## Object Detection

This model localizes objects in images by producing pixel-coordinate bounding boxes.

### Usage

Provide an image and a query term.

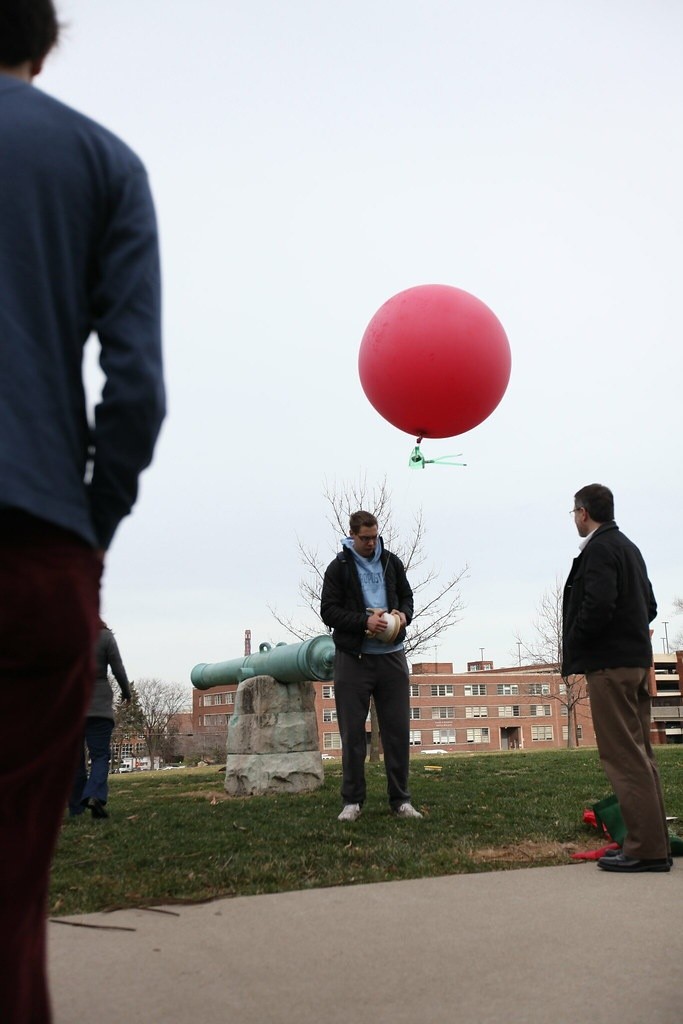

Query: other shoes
[91,802,108,820]
[596,854,670,872]
[605,849,673,866]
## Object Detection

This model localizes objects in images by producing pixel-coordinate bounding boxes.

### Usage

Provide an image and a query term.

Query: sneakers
[397,802,423,819]
[338,803,361,822]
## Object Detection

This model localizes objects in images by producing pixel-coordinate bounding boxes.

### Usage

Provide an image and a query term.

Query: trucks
[118,756,186,773]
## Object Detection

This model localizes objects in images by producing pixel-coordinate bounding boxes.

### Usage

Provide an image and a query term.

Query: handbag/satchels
[592,794,628,848]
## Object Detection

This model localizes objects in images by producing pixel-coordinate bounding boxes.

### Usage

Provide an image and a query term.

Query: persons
[560,484,673,872]
[67,617,131,818]
[0,0,167,1023]
[321,510,424,821]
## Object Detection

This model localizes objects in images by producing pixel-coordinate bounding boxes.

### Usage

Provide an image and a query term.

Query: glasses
[572,505,587,517]
[354,531,377,541]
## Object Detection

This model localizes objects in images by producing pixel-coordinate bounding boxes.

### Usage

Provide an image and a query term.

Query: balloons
[359,284,511,443]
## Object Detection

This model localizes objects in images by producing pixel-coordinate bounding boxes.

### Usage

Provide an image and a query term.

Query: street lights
[660,638,666,654]
[662,621,670,654]
[479,648,485,670]
[516,642,523,667]
